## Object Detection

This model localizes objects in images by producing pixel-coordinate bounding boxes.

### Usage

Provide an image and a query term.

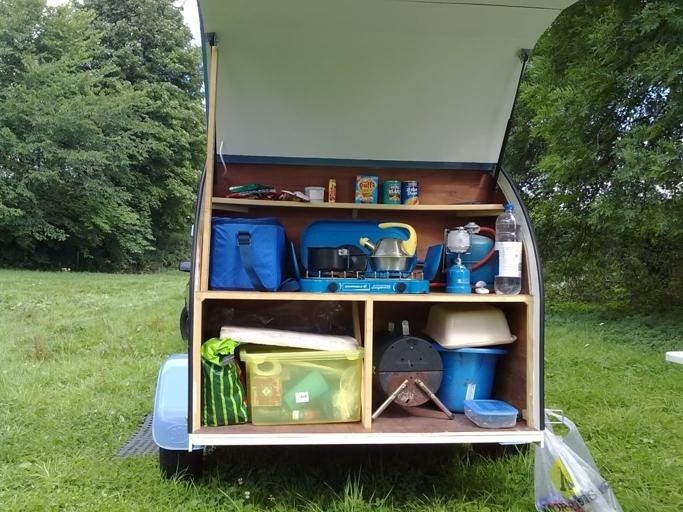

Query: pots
[305,246,350,273]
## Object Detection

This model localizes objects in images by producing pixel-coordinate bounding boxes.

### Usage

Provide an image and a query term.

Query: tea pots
[360,221,417,272]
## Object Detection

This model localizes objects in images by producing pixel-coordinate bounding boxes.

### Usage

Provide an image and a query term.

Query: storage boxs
[239,349,365,427]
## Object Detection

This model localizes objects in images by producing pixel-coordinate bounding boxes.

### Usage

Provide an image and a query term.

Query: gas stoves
[299,268,430,295]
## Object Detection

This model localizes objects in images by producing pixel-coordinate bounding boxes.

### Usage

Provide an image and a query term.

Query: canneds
[400,181,419,205]
[383,180,402,204]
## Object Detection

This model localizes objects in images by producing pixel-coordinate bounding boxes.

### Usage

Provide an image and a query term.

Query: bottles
[326,175,337,203]
[252,407,299,423]
[491,200,523,295]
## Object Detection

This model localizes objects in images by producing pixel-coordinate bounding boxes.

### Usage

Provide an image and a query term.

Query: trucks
[149,0,578,478]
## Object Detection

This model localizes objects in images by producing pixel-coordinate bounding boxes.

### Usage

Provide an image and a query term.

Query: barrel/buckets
[431,343,506,414]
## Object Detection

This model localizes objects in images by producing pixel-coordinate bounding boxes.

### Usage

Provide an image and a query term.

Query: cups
[282,371,329,409]
[303,186,325,202]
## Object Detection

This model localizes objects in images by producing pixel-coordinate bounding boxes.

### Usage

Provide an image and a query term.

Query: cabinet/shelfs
[188,157,549,446]
